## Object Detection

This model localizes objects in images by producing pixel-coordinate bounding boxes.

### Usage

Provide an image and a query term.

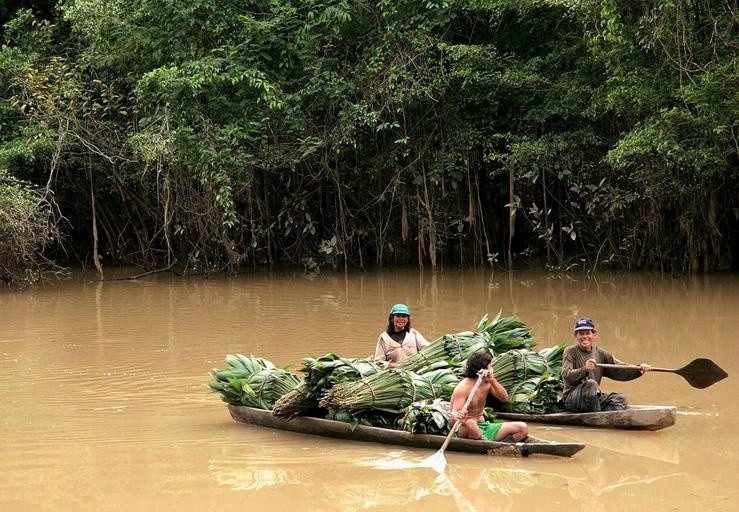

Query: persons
[374,303,431,368]
[448,351,528,442]
[562,317,651,412]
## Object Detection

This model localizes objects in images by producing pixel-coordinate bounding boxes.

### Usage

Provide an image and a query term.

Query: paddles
[591,358,729,389]
[420,370,490,468]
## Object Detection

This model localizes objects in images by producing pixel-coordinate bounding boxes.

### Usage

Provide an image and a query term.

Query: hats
[575,317,595,331]
[390,304,410,315]
